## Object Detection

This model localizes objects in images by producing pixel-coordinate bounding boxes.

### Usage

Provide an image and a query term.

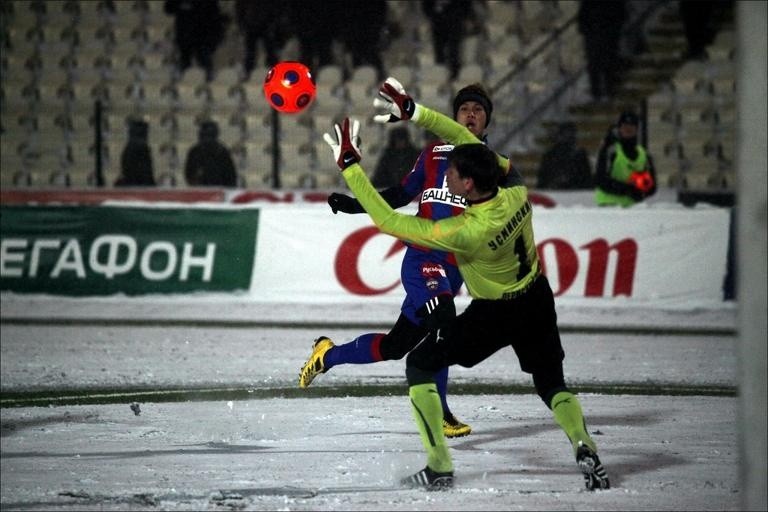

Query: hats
[617,110,639,126]
[452,81,493,131]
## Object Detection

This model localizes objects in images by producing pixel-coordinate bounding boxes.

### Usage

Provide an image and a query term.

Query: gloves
[327,186,362,220]
[322,115,364,173]
[370,75,416,125]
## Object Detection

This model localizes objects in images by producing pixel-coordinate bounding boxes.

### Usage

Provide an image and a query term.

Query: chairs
[0,0,587,191]
[647,28,736,196]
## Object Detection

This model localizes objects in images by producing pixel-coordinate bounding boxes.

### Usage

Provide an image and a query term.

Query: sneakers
[440,417,473,440]
[399,464,455,491]
[296,335,336,391]
[574,443,611,492]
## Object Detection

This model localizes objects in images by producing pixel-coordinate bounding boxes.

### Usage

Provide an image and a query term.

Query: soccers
[263,60,316,115]
[627,170,656,193]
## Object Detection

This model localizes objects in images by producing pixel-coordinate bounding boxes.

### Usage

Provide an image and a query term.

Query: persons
[298,85,493,438]
[322,76,610,491]
